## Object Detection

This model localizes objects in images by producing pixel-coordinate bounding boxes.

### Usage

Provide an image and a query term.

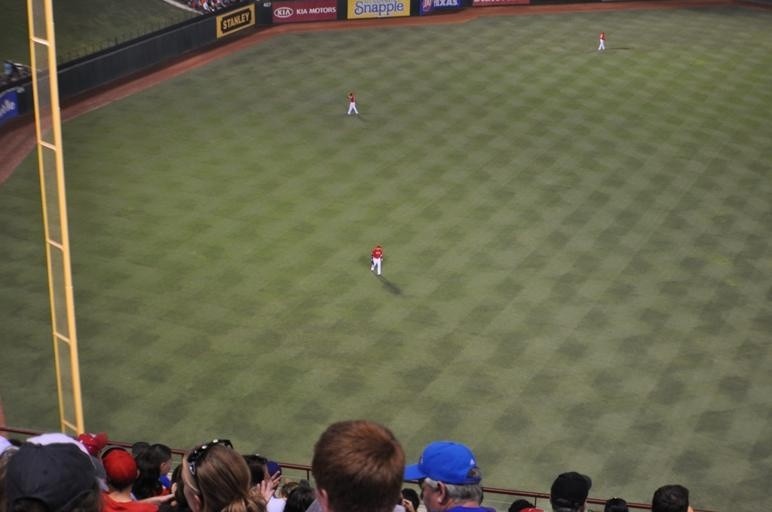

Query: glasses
[187,438,233,504]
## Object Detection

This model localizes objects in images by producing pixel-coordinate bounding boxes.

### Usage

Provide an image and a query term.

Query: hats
[102,448,137,483]
[404,441,482,485]
[6,433,107,511]
[78,432,108,455]
[266,461,281,478]
[551,474,591,507]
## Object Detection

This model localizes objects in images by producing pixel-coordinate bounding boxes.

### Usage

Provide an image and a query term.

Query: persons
[189,1,243,15]
[347,92,359,116]
[370,245,383,275]
[598,32,606,51]
